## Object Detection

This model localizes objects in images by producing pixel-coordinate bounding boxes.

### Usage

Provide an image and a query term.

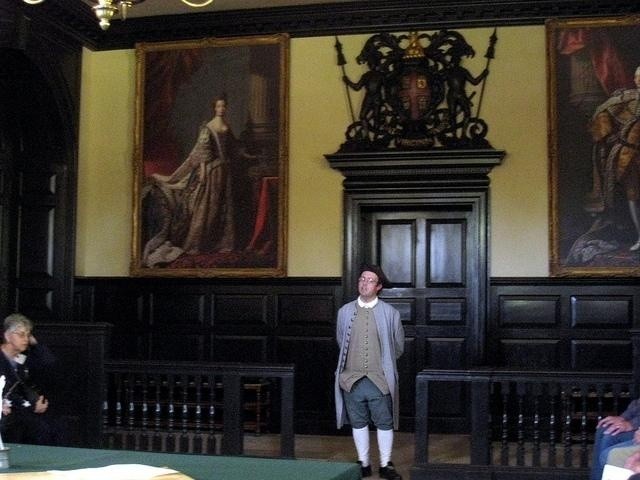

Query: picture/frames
[130,33,290,279]
[544,15,640,278]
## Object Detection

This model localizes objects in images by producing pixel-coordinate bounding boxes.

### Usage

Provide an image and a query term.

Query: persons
[590,399,640,480]
[559,68,640,267]
[432,56,489,146]
[343,55,394,145]
[334,265,405,480]
[138,94,271,268]
[1,315,70,447]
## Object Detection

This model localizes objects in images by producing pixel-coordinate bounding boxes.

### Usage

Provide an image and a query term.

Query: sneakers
[379,461,402,479]
[356,460,372,477]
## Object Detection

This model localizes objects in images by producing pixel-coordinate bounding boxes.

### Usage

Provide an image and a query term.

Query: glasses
[359,276,374,283]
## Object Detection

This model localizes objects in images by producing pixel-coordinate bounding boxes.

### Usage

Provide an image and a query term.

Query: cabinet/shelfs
[123,379,270,437]
[559,390,631,445]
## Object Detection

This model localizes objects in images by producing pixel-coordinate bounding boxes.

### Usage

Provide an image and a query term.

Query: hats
[360,265,384,282]
